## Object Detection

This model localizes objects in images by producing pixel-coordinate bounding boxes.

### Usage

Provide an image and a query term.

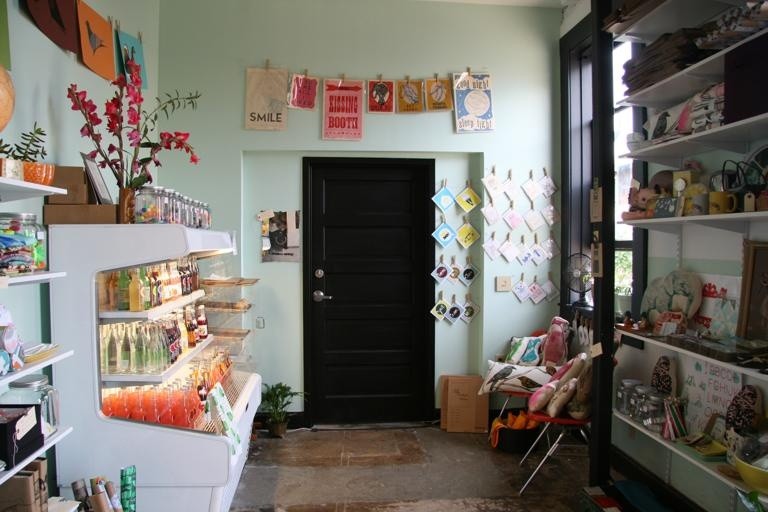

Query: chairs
[488,329,548,440]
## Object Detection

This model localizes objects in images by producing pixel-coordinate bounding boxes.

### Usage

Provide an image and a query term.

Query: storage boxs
[40,165,119,228]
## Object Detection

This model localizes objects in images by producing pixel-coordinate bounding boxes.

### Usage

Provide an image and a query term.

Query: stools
[513,406,590,495]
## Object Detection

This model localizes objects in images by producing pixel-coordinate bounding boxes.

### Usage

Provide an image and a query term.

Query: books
[0,457,49,511]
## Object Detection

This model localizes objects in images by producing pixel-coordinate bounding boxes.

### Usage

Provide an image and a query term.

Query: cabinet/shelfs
[1,177,82,512]
[45,221,265,511]
[597,0,768,511]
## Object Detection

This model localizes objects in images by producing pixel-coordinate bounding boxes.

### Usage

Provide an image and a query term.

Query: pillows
[477,317,589,417]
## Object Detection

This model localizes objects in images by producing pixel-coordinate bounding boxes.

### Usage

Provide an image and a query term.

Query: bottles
[101,345,232,427]
[616,378,672,432]
[134,185,212,229]
[0,374,60,439]
[108,256,203,312]
[100,305,214,373]
[0,210,47,269]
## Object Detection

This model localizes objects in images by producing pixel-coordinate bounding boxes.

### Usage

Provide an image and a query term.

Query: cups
[708,190,737,215]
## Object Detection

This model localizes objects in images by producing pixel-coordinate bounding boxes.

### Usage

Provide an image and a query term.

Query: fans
[561,248,594,306]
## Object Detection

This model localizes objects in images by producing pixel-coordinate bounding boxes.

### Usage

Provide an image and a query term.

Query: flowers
[66,54,203,195]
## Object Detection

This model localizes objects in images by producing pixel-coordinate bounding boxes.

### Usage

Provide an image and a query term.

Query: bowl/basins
[732,451,768,496]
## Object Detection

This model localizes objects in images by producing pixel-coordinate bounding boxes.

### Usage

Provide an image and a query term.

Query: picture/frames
[730,234,768,350]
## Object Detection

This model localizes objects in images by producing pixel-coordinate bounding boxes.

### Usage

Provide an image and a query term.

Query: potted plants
[256,378,310,436]
[0,117,57,185]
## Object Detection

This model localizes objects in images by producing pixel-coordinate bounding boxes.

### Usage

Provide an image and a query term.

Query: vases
[118,192,135,224]
